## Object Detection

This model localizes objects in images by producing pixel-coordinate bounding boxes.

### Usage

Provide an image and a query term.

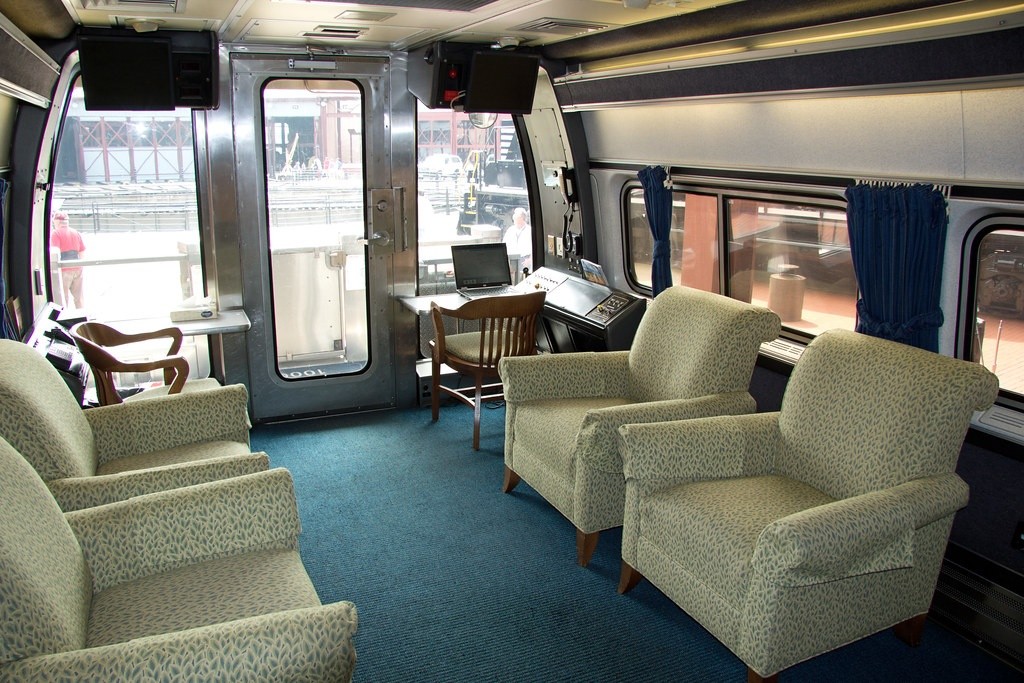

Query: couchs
[1,337,273,514]
[496,281,781,575]
[616,327,1001,681]
[1,431,360,683]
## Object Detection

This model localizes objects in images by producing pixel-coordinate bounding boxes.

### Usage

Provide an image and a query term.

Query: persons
[51,212,86,310]
[502,208,533,274]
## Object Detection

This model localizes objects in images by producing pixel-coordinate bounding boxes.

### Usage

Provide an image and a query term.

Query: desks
[164,308,254,384]
[398,285,524,321]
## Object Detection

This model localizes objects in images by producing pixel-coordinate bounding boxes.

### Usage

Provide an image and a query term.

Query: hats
[52,210,70,220]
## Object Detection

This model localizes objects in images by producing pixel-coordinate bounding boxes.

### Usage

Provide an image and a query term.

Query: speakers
[81,28,219,108]
[407,41,534,109]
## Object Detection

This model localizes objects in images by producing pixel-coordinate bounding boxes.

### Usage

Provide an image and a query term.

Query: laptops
[451,242,527,302]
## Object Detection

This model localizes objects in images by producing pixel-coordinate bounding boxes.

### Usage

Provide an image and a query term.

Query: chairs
[68,320,252,429]
[425,290,555,455]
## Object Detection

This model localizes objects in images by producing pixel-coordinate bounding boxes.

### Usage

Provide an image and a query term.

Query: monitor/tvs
[78,38,172,111]
[464,48,540,114]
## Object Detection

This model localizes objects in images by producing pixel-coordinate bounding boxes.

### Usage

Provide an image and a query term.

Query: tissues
[171,294,220,323]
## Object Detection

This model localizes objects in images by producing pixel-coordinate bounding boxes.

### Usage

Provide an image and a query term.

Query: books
[580,259,608,286]
[5,296,24,341]
